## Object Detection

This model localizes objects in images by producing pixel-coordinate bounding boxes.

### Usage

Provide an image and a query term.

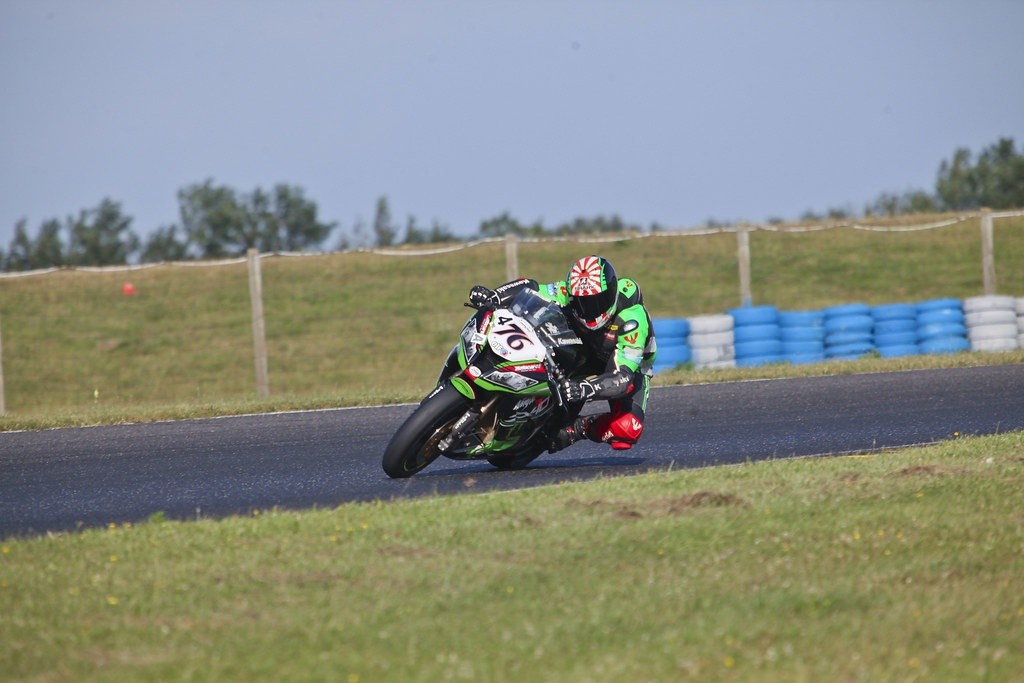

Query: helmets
[567,255,617,330]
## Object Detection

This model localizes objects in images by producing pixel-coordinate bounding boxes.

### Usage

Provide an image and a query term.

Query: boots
[554,412,608,449]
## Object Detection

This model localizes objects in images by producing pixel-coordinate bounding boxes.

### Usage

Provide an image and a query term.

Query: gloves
[469,286,498,311]
[560,378,594,403]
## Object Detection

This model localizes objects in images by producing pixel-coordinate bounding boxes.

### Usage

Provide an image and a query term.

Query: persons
[470,254,657,455]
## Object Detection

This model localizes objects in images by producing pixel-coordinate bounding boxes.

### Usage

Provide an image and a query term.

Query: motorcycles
[380,277,596,481]
